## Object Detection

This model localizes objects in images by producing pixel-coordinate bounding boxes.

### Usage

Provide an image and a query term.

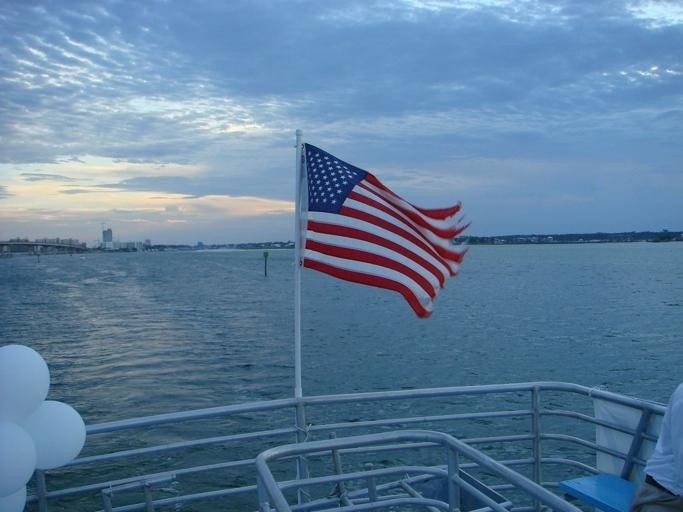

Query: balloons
[0,421,37,497]
[0,486,27,512]
[22,401,86,470]
[0,344,50,422]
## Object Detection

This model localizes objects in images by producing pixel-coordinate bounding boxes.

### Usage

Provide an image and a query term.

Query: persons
[630,383,683,512]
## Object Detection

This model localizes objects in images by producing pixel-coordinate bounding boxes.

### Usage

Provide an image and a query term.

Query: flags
[298,143,473,319]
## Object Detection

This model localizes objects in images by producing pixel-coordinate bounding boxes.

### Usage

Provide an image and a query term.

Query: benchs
[558,473,637,512]
